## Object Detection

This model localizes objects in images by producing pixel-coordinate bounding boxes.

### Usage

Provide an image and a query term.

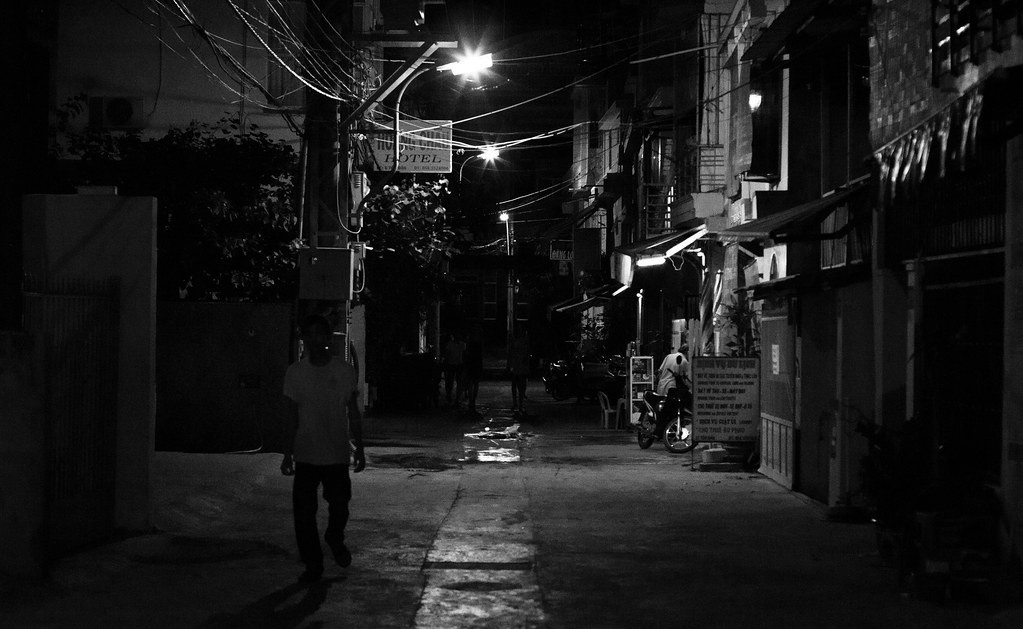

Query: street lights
[343,34,499,361]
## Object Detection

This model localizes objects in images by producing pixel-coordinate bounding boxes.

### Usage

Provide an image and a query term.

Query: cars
[479,335,511,379]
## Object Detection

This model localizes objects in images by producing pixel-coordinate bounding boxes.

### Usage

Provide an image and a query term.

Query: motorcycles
[543,347,630,405]
[631,356,699,453]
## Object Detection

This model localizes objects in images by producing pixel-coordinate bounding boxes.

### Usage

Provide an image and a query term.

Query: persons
[455,335,481,415]
[439,332,466,410]
[509,324,529,412]
[657,343,691,397]
[281,314,366,581]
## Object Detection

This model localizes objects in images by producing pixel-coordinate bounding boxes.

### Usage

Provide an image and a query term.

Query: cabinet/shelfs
[629,355,655,424]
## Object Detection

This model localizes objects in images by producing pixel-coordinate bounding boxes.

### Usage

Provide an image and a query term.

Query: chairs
[597,391,617,429]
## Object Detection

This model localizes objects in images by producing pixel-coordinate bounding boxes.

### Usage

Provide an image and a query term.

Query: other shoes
[298,565,324,582]
[330,543,352,568]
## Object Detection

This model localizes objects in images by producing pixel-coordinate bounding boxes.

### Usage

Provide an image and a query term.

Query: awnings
[616,223,708,258]
[716,179,870,242]
[556,295,612,313]
[732,273,802,293]
